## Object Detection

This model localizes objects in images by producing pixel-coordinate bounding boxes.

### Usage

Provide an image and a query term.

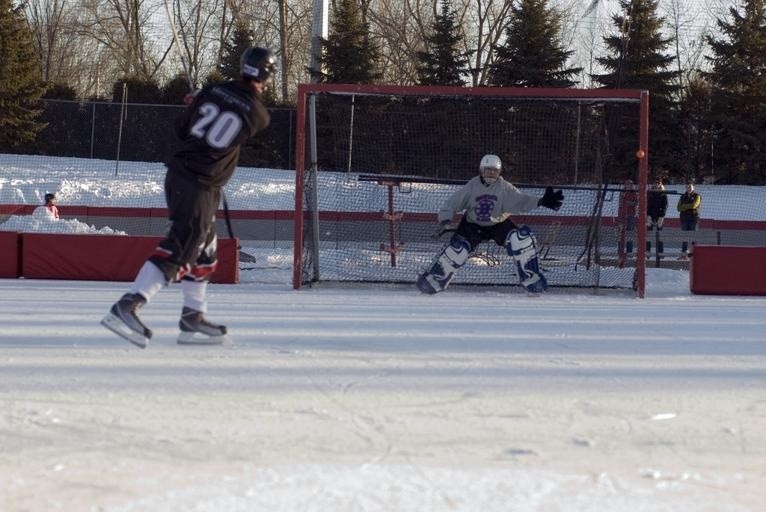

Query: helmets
[239,47,279,83]
[45,194,55,201]
[480,155,502,185]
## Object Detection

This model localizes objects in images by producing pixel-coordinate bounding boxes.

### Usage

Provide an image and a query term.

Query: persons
[109,47,282,340]
[645,175,669,258]
[41,194,61,220]
[417,154,564,296]
[617,179,639,257]
[677,180,702,259]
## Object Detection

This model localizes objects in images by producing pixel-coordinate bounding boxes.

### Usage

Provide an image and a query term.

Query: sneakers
[179,306,226,336]
[111,293,152,339]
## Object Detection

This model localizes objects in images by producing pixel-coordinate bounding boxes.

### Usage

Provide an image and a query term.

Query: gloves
[432,220,458,240]
[537,186,564,212]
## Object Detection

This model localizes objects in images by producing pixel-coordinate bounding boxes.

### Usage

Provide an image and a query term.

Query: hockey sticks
[222,187,256,262]
[577,182,608,266]
